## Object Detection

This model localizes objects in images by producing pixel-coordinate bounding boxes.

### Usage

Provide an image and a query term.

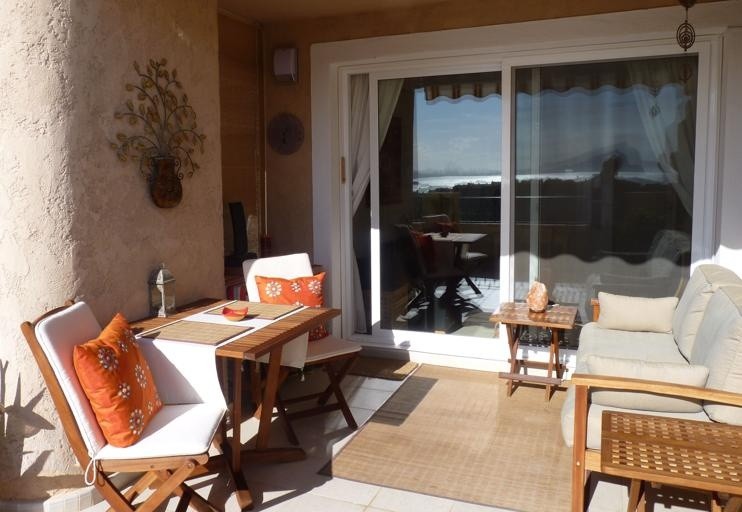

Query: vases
[151,157,182,208]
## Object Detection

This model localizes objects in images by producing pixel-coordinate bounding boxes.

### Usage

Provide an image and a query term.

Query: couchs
[585,229,691,323]
[560,263,742,512]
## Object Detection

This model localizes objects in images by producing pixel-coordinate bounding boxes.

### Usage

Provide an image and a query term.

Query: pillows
[588,356,709,413]
[596,291,679,334]
[255,271,329,342]
[73,313,163,449]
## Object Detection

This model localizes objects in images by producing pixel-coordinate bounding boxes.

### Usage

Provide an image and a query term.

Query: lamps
[272,47,298,82]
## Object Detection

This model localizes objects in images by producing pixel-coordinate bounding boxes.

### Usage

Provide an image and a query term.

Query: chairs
[423,214,487,294]
[242,251,363,456]
[21,297,228,512]
[393,224,463,309]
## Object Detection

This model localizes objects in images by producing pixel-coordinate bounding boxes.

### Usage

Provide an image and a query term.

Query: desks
[426,232,487,298]
[489,302,578,402]
[601,409,742,510]
[127,298,343,512]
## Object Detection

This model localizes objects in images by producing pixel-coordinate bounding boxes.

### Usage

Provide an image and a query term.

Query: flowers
[110,57,207,179]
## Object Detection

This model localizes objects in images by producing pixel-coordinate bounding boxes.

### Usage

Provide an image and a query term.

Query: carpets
[317,362,592,512]
[350,356,419,381]
